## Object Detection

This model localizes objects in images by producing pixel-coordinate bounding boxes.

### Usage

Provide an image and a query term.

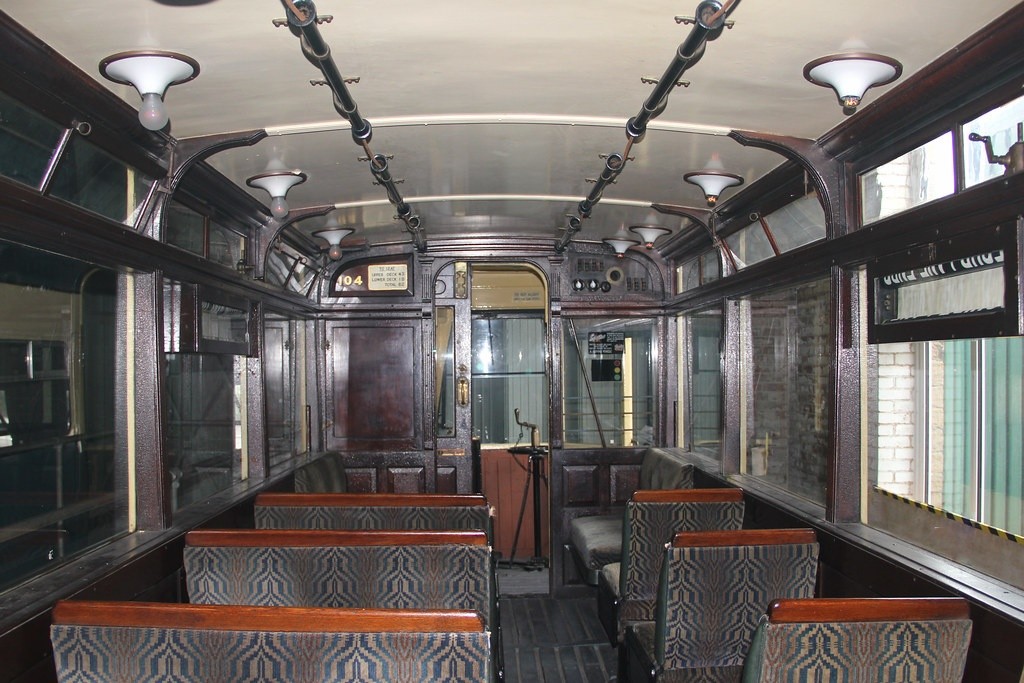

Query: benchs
[183,527,500,662]
[50,599,501,683]
[252,490,495,557]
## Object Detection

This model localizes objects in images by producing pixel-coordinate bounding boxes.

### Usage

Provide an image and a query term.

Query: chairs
[569,444,695,586]
[741,597,972,683]
[597,485,746,649]
[616,527,820,683]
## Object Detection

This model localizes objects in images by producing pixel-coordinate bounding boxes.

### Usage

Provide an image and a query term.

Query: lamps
[803,51,904,116]
[604,237,642,259]
[98,49,201,131]
[629,224,673,250]
[246,170,308,219]
[683,170,744,208]
[311,227,356,261]
